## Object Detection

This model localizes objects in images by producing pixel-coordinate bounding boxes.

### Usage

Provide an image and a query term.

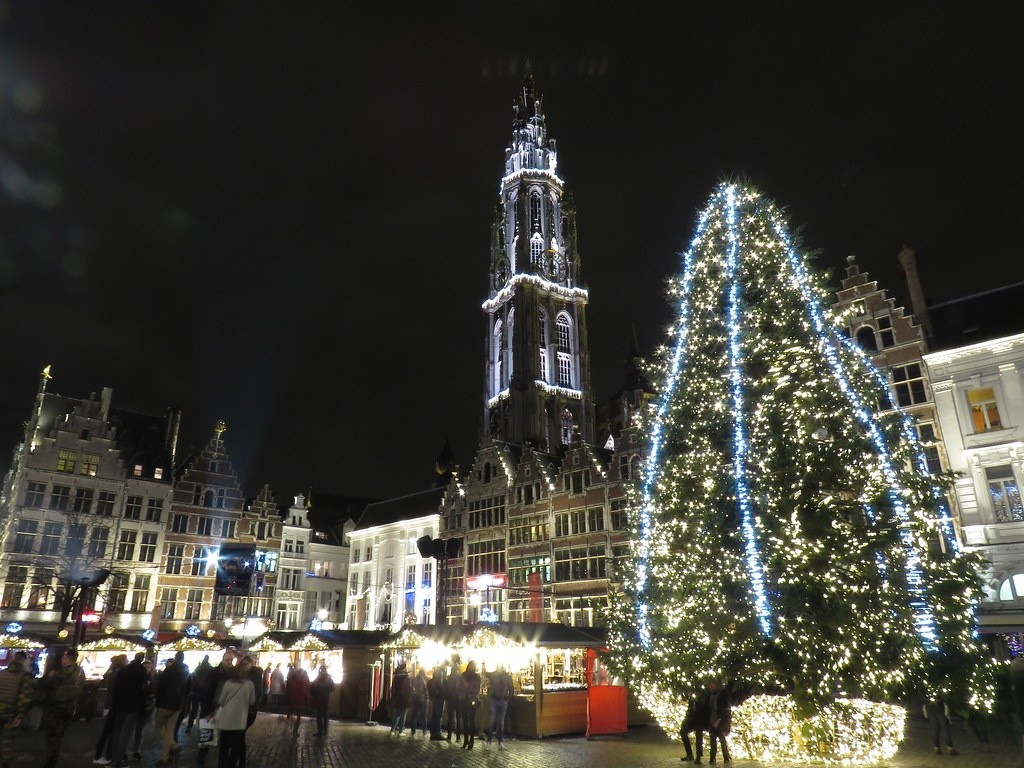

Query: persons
[0,650,85,768]
[390,661,481,750]
[310,666,335,736]
[922,695,955,754]
[680,679,732,764]
[969,710,991,752]
[481,656,514,751]
[92,650,310,768]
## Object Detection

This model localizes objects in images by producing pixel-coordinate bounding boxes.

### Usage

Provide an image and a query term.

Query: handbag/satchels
[468,695,482,711]
[197,715,218,748]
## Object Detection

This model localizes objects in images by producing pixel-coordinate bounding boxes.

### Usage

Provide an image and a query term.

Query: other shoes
[467,740,474,749]
[92,757,112,765]
[133,753,144,761]
[398,732,407,737]
[456,734,461,742]
[695,755,700,764]
[948,748,960,755]
[498,742,507,750]
[724,756,731,763]
[313,729,327,736]
[935,748,942,755]
[461,739,471,748]
[681,754,694,761]
[430,733,446,741]
[446,734,451,741]
[709,757,716,764]
[485,743,491,750]
[389,731,395,736]
